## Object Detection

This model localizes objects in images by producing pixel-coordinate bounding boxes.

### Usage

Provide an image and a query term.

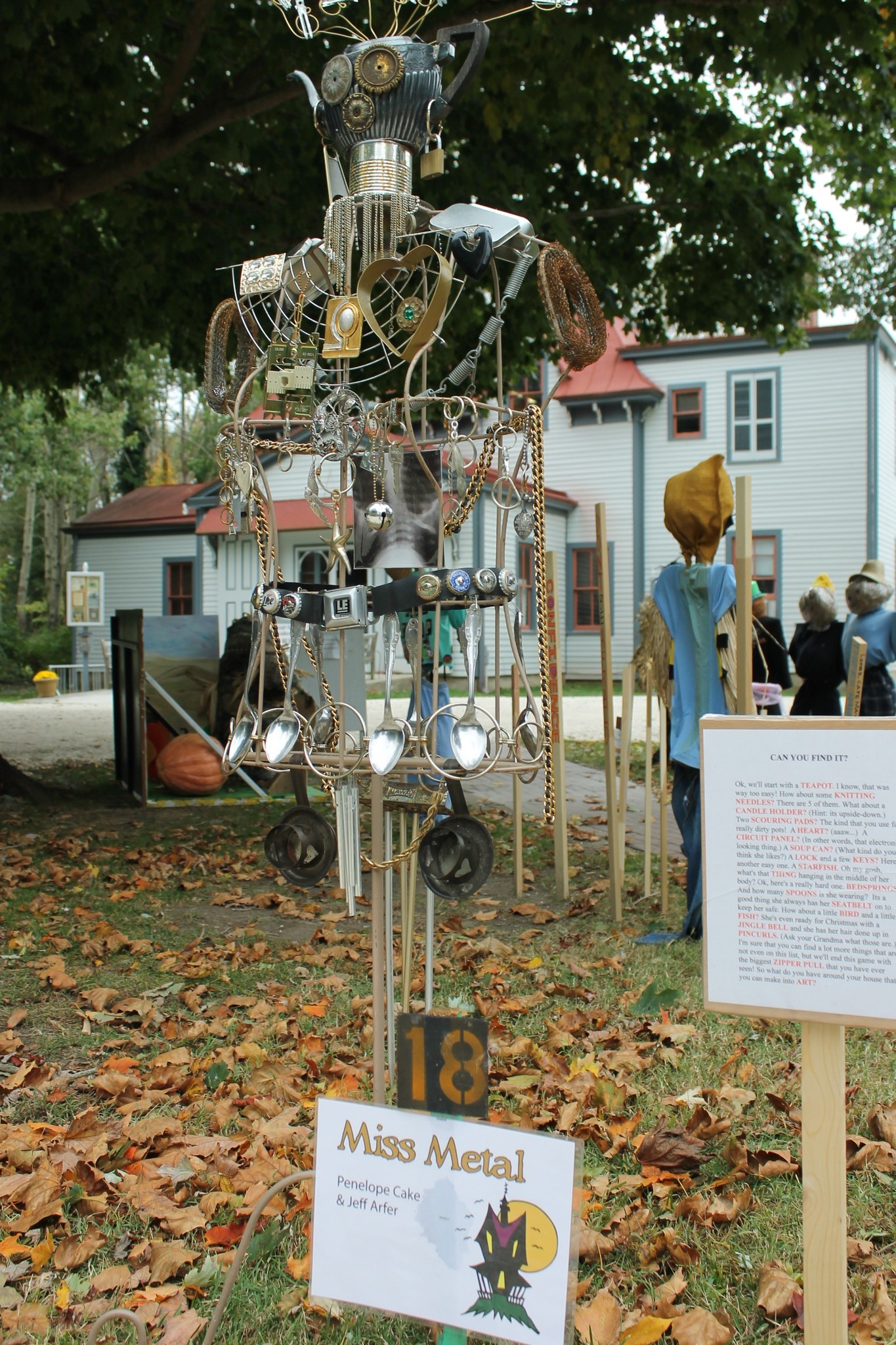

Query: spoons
[450,605,488,769]
[402,618,432,759]
[264,589,306,763]
[368,610,405,776]
[457,620,490,759]
[228,610,262,763]
[513,611,537,760]
[310,622,340,755]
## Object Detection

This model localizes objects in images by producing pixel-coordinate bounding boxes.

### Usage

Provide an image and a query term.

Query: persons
[632,456,755,945]
[751,580,793,715]
[788,574,848,716]
[840,559,896,716]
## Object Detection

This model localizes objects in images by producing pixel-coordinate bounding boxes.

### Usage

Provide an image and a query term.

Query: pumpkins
[156,734,229,794]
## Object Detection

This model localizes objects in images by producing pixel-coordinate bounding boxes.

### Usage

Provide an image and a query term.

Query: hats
[849,559,887,584]
[751,580,766,601]
